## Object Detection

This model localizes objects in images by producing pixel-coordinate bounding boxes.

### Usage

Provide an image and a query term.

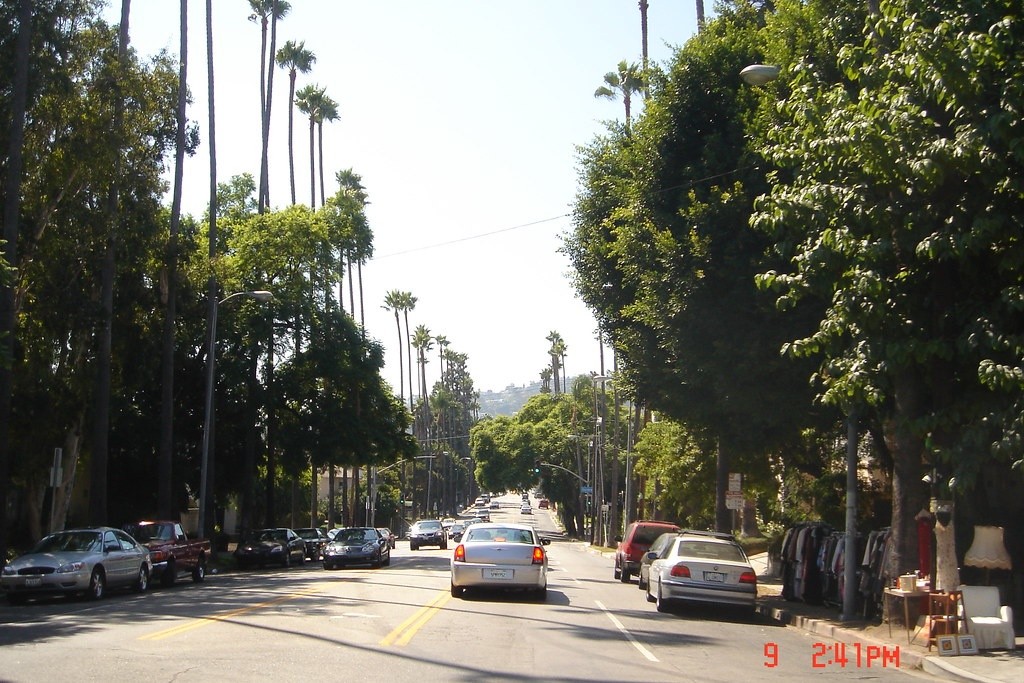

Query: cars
[449,523,552,597]
[409,520,446,550]
[282,527,330,560]
[231,527,309,569]
[448,491,500,542]
[614,519,682,583]
[1,523,157,598]
[521,487,549,514]
[373,528,396,549]
[637,527,760,615]
[322,527,391,567]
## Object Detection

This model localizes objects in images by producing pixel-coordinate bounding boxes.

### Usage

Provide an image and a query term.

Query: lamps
[963,526,1012,586]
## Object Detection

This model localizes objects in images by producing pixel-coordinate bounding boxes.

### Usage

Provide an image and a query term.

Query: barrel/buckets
[900,575,916,591]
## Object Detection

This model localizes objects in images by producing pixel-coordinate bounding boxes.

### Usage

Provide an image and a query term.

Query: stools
[928,590,970,652]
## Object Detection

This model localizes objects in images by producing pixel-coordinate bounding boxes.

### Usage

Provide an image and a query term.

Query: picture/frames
[936,635,959,656]
[957,634,978,655]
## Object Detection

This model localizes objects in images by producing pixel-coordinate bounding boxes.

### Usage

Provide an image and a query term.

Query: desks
[885,587,931,644]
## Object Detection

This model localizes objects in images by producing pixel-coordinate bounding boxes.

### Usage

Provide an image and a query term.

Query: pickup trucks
[109,519,213,585]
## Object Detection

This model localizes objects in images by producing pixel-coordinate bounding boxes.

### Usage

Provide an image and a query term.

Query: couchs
[962,585,1016,649]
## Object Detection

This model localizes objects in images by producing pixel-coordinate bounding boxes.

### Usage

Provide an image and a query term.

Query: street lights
[364,454,440,527]
[540,432,590,541]
[196,288,276,535]
[739,61,858,616]
[594,375,635,534]
